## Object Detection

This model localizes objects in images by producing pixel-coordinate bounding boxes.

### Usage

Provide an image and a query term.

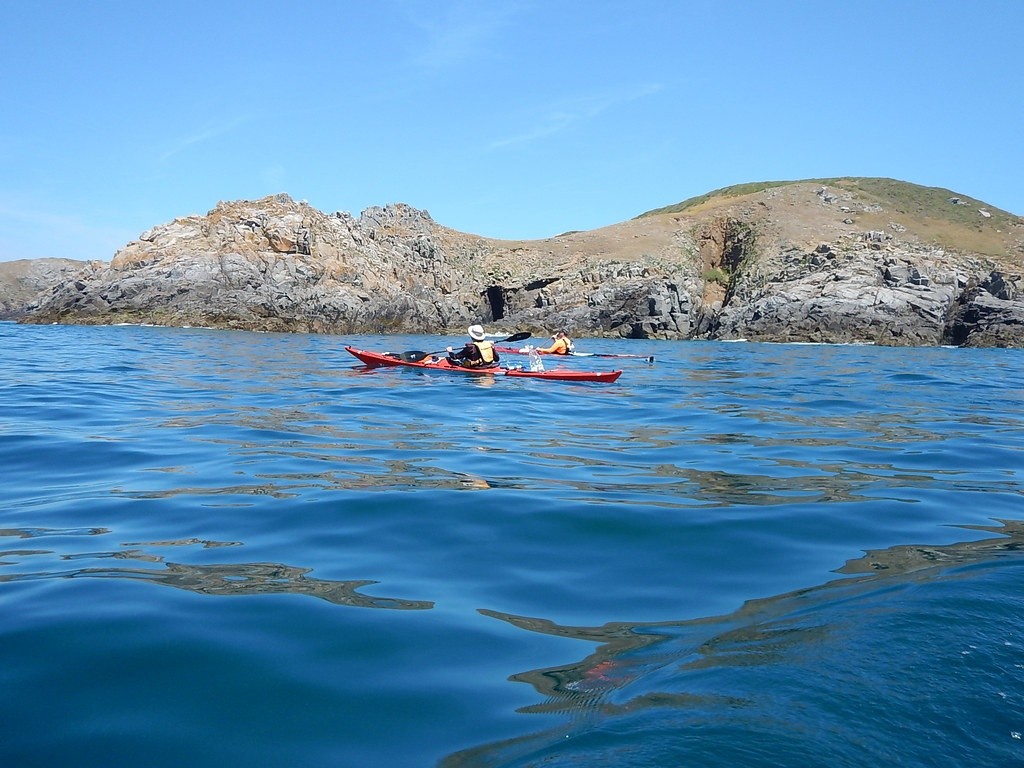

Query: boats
[464,343,648,359]
[344,346,624,383]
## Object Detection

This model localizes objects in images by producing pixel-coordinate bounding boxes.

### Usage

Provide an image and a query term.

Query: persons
[446,324,499,365]
[536,328,575,357]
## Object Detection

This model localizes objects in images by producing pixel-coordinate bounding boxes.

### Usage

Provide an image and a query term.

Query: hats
[467,324,486,341]
[557,328,569,335]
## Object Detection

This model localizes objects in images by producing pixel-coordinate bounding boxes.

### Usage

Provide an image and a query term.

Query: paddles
[533,331,560,352]
[399,332,532,362]
